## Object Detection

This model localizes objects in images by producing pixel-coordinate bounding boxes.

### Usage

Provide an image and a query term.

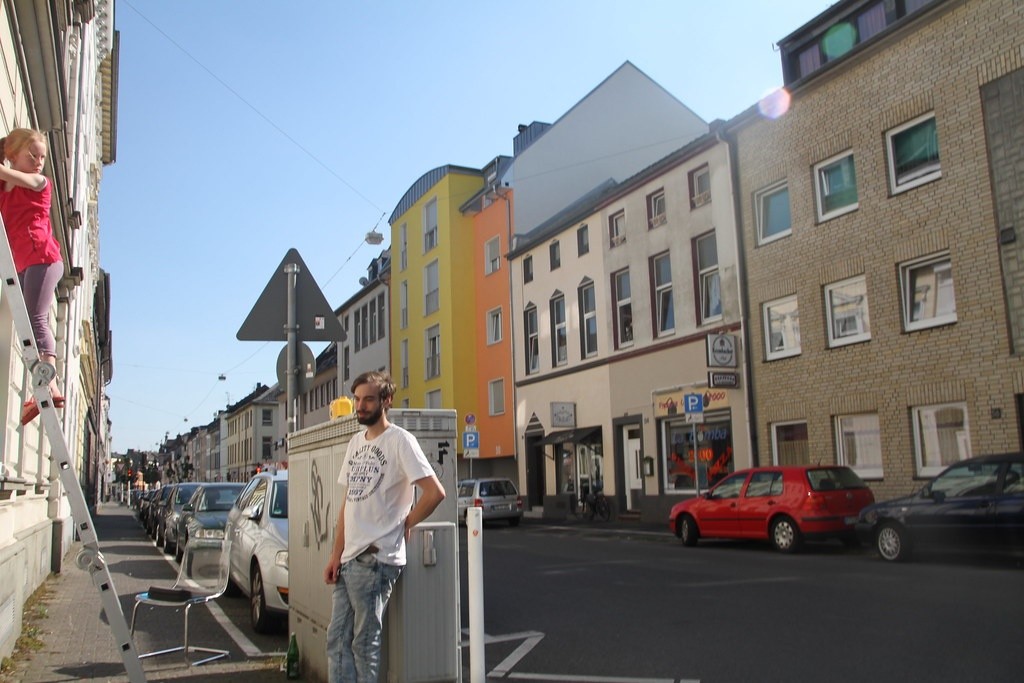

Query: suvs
[223,466,288,633]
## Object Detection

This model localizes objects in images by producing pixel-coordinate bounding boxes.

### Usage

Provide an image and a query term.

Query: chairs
[820,478,835,490]
[990,471,1021,494]
[130,539,234,664]
[178,489,192,503]
[206,491,225,510]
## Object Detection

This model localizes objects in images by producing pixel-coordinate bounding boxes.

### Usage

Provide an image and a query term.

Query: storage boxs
[147,587,192,604]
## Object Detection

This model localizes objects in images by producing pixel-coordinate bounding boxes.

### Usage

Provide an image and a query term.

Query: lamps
[365,230,384,245]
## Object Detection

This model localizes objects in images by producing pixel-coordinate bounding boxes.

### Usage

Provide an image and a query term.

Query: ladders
[0,212,154,683]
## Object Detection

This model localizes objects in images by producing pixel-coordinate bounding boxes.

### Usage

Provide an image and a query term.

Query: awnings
[535,426,605,458]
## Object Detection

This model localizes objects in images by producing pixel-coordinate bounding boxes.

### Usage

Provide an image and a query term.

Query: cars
[669,464,877,554]
[855,451,1024,563]
[457,477,522,527]
[175,483,248,565]
[138,482,206,553]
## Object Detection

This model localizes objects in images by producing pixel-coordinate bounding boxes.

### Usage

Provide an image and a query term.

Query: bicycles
[573,486,612,523]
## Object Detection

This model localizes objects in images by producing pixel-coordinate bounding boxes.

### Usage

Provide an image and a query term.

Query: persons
[0,128,67,426]
[322,370,447,683]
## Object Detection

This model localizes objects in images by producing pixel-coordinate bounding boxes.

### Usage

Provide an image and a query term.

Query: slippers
[20,396,64,425]
[53,401,64,408]
